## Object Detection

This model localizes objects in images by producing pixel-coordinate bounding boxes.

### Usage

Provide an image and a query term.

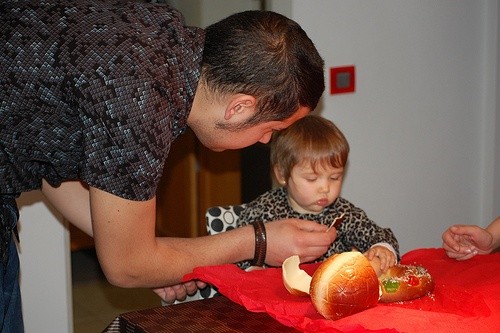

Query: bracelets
[246,219,269,270]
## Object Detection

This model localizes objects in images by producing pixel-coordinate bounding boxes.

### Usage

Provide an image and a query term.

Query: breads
[309,251,380,321]
[377,264,433,303]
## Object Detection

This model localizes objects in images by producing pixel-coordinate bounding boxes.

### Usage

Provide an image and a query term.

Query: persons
[237,114,403,269]
[441,217,500,261]
[0,0,335,333]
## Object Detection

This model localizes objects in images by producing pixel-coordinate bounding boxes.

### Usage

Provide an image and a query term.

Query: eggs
[366,255,382,277]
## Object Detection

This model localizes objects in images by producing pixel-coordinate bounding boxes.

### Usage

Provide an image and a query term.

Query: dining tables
[100,248,500,333]
